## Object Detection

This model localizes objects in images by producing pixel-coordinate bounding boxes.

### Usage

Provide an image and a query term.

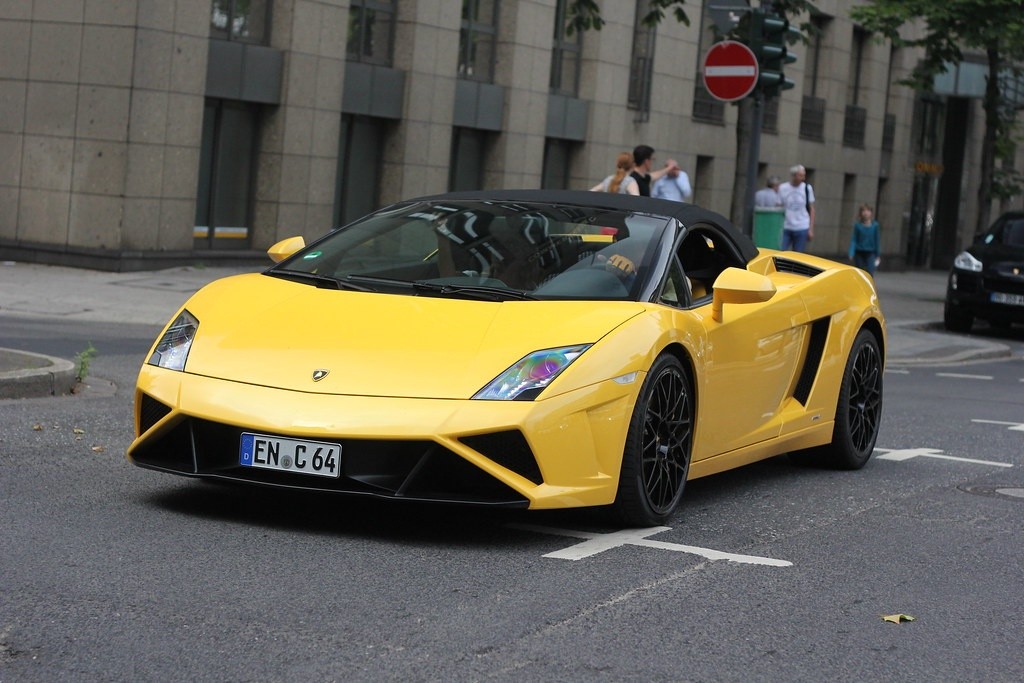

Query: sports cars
[124,191,890,527]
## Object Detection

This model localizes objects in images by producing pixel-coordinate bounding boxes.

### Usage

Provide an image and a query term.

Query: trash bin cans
[750,207,787,251]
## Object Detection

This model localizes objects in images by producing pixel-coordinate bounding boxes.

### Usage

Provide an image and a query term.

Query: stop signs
[702,41,758,103]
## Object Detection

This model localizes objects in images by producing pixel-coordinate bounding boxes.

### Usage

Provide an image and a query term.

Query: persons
[848,203,881,277]
[589,145,692,203]
[579,214,692,306]
[753,177,781,208]
[777,163,816,254]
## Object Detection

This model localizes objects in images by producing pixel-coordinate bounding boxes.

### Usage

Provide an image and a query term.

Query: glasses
[647,156,657,162]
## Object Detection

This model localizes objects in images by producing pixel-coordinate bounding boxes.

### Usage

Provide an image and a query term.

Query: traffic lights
[755,13,799,98]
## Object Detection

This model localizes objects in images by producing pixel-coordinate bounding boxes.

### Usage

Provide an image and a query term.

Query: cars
[942,209,1024,338]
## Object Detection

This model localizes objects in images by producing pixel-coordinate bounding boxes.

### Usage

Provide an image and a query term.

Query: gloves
[597,254,637,282]
